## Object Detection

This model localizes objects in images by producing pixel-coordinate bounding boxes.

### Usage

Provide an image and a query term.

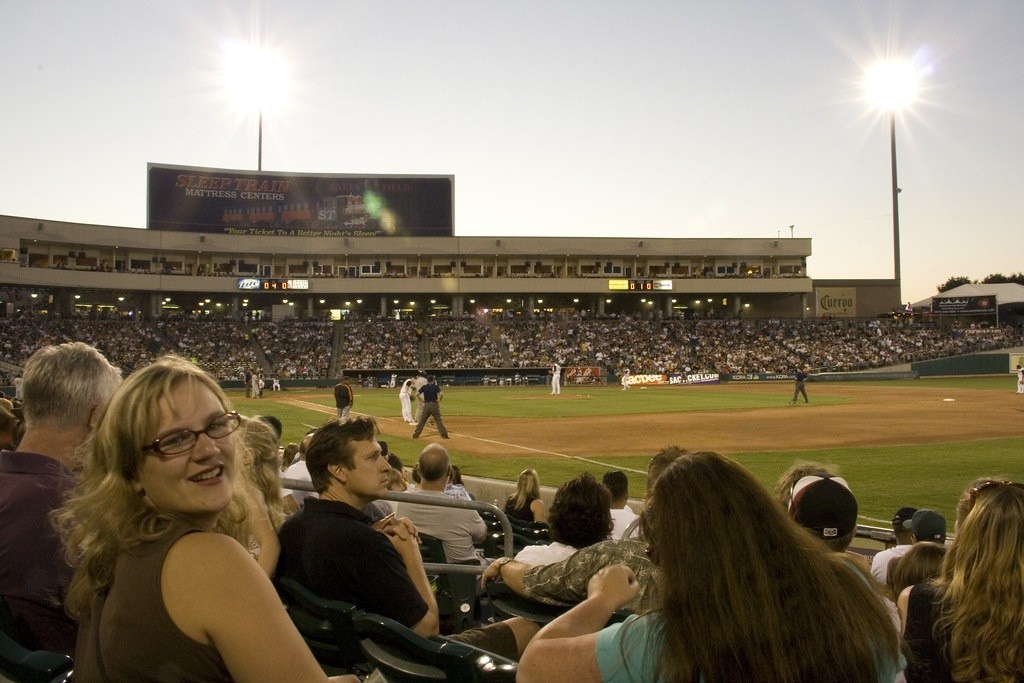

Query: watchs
[498,557,514,579]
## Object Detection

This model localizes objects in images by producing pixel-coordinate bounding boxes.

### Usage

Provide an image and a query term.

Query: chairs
[0,514,635,683]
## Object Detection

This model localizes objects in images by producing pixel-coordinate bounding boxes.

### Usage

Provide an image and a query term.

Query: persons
[514,452,906,683]
[0,309,1024,683]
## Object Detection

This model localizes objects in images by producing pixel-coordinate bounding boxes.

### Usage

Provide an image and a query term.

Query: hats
[786,475,858,540]
[902,509,945,538]
[892,507,919,528]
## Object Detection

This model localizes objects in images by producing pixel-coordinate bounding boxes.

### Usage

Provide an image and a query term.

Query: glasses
[143,412,241,455]
[969,478,1024,510]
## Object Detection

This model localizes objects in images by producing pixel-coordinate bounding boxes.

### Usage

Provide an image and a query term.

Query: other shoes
[441,435,450,439]
